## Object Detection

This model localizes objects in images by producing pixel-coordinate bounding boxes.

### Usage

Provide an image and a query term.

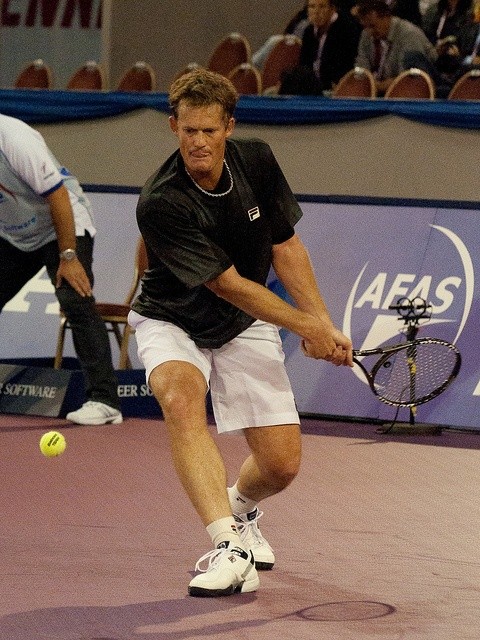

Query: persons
[357,4,437,92]
[428,0,473,63]
[124,73,354,597]
[432,30,479,98]
[277,0,357,97]
[0,115,125,426]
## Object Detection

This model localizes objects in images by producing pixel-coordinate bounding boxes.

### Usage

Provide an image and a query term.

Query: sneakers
[66,401,123,425]
[232,505,275,570]
[188,540,260,597]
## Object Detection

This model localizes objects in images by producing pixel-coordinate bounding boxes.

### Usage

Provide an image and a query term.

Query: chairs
[207,32,251,76]
[114,62,156,92]
[224,63,262,95]
[53,237,148,374]
[383,68,436,100]
[63,63,107,92]
[446,70,480,99]
[263,36,302,91]
[331,68,375,99]
[13,60,53,88]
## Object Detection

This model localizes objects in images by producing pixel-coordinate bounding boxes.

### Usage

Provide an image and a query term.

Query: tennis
[40,431,66,457]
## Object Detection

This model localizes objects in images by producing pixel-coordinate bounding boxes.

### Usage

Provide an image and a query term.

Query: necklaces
[180,159,234,198]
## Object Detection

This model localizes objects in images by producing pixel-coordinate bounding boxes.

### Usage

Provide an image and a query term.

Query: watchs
[59,249,76,261]
